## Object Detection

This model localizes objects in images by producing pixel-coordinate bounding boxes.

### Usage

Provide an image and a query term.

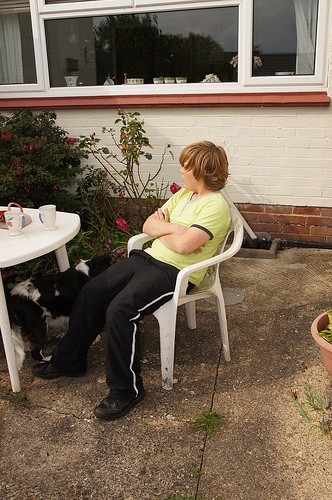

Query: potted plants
[311,308,332,376]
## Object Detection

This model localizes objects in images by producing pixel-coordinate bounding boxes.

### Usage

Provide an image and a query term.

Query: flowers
[0,131,182,259]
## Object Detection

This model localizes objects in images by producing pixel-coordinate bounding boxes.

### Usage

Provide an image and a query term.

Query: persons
[30,141,232,422]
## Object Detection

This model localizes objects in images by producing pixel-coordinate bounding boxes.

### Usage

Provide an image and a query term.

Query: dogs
[6,254,116,371]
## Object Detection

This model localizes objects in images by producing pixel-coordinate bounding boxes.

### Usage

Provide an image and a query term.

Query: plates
[127,78,144,84]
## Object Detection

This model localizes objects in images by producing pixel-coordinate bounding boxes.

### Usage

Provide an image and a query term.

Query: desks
[0,206,81,394]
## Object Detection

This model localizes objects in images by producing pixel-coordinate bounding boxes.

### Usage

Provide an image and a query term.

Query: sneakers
[32,363,86,379]
[94,389,146,419]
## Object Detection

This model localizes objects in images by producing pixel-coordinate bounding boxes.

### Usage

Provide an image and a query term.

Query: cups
[176,77,187,83]
[154,78,164,84]
[38,205,56,228]
[165,77,175,83]
[4,209,23,236]
[64,75,78,86]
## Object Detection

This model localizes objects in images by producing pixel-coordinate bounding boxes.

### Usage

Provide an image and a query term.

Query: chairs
[127,207,244,391]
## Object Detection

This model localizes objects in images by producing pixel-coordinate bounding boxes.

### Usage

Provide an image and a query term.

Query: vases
[63,76,79,86]
